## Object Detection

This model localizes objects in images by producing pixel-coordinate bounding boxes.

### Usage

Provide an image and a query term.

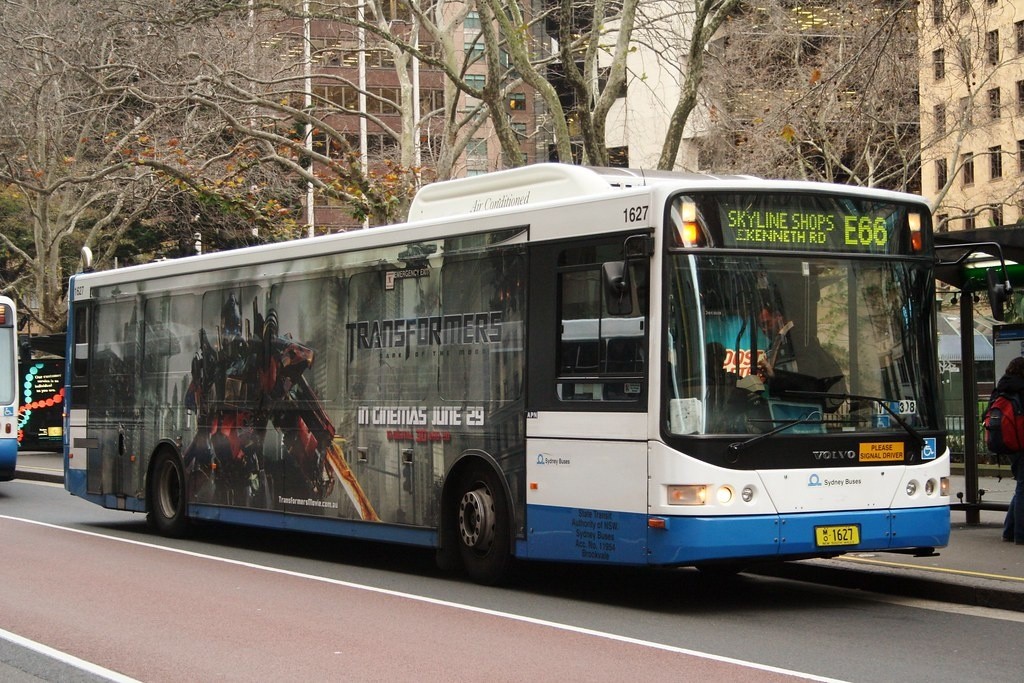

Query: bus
[60,163,957,583]
[0,296,20,478]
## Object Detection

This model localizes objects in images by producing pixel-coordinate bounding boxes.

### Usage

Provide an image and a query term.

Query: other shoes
[1002,536,1024,545]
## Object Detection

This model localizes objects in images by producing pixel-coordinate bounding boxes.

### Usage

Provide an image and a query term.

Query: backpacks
[984,381,1024,455]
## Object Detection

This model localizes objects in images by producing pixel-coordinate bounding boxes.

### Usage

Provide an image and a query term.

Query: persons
[757,304,825,421]
[701,339,745,405]
[987,358,1024,544]
